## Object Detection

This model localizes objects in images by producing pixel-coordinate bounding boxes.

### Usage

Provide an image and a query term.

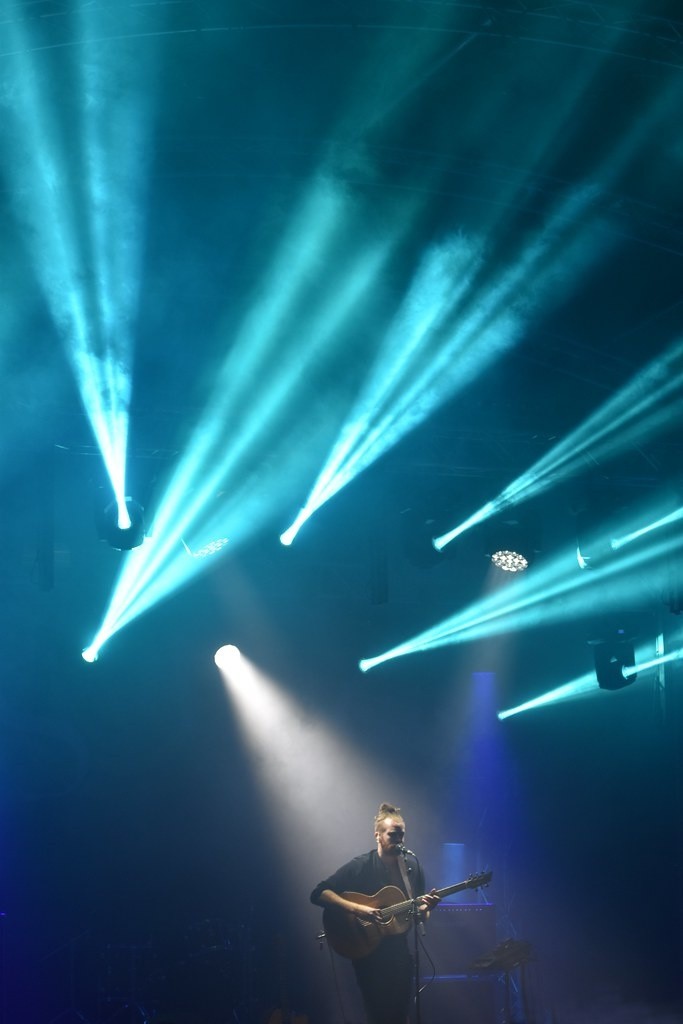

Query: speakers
[408,976,498,1024]
[413,903,499,977]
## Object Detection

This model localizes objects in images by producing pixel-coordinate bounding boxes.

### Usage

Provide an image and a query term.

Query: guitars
[321,868,495,958]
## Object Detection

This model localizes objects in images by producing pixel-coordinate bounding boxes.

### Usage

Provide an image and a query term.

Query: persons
[309,803,441,1023]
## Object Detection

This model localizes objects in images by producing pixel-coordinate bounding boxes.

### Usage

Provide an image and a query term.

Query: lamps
[593,627,637,691]
[90,468,154,549]
[491,536,534,573]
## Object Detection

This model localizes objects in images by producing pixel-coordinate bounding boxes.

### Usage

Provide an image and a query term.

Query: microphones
[396,843,414,856]
